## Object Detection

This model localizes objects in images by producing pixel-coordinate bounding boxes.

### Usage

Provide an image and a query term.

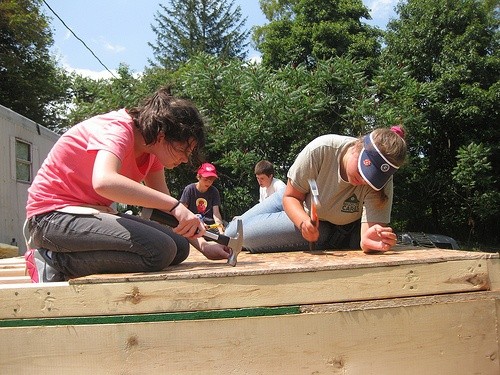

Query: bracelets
[300,221,303,232]
[169,200,181,213]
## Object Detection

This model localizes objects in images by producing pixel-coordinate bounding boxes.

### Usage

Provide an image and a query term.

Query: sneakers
[24,249,66,283]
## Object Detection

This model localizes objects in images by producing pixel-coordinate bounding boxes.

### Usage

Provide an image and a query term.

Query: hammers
[142,208,244,267]
[306,203,319,250]
[203,223,223,229]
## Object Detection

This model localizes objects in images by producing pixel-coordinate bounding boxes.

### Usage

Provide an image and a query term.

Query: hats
[358,130,400,191]
[197,163,218,178]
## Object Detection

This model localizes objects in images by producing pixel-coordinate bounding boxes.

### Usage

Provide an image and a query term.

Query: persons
[255,160,287,203]
[24,88,233,284]
[181,161,229,234]
[225,125,407,253]
